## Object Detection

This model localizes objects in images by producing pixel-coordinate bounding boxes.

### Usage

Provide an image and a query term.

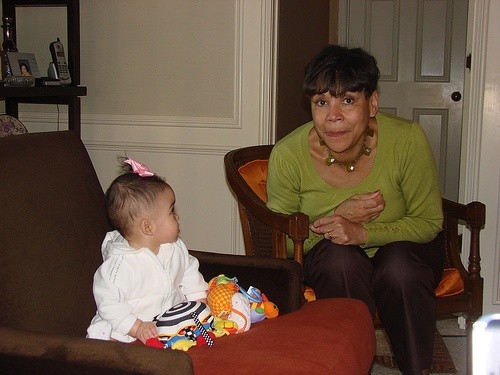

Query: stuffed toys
[146,274,278,351]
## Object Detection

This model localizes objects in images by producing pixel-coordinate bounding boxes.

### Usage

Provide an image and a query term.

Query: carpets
[366,321,457,375]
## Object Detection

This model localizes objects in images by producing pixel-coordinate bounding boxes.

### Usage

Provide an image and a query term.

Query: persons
[86,156,209,344]
[266,44,445,375]
[20,63,32,76]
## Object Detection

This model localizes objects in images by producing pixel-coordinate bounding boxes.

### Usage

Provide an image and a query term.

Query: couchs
[0,130,376,375]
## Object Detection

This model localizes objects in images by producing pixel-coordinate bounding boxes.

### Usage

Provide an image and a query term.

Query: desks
[0,86,88,138]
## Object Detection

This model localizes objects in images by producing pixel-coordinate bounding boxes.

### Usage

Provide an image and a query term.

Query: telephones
[47,37,73,85]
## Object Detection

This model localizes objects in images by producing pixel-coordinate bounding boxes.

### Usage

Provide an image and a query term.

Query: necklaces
[320,126,374,173]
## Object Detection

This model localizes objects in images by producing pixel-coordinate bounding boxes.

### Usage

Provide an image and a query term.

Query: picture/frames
[8,52,41,78]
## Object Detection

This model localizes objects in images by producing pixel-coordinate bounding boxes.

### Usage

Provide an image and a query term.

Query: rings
[328,232,331,238]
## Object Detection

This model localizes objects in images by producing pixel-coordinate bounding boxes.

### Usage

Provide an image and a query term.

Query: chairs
[224,145,486,375]
